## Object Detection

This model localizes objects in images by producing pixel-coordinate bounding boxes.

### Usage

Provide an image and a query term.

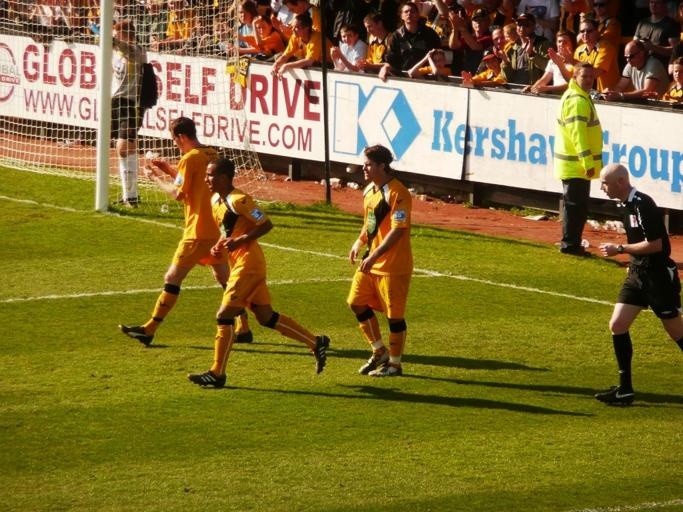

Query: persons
[629,0,679,62]
[87,22,158,209]
[378,0,620,96]
[358,16,395,77]
[188,157,331,388]
[328,27,371,75]
[552,62,602,258]
[227,0,329,80]
[662,57,683,103]
[594,163,683,404]
[346,145,414,377]
[0,1,234,52]
[602,41,668,101]
[119,117,254,347]
[326,0,386,42]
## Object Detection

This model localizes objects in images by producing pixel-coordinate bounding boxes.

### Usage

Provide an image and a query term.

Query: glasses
[580,29,596,33]
[594,2,608,7]
[624,50,643,59]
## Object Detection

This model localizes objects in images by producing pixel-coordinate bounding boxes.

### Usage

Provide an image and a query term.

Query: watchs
[619,93,624,99]
[618,245,624,254]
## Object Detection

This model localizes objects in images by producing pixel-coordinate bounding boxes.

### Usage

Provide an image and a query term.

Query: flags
[227,56,249,88]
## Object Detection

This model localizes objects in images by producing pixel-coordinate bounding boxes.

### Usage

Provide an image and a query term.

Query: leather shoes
[560,245,585,255]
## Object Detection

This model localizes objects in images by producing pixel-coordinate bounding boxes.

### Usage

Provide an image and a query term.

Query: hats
[513,13,536,23]
[472,8,490,20]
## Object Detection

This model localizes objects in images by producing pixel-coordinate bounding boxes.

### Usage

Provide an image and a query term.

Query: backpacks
[140,62,157,109]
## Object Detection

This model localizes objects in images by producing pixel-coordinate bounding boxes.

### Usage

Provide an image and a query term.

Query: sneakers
[119,324,154,346]
[121,198,137,208]
[595,387,634,405]
[312,334,331,374]
[187,370,226,387]
[119,196,141,203]
[369,362,402,377]
[232,331,253,343]
[359,352,389,375]
